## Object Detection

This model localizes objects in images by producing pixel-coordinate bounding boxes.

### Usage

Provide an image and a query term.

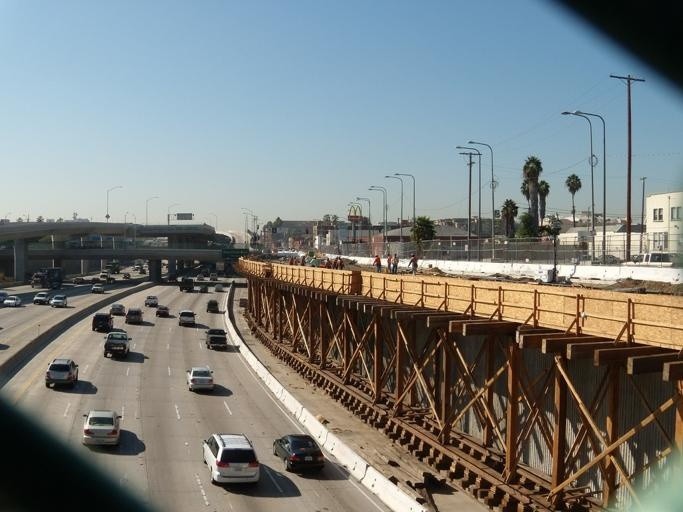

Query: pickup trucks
[619,251,683,267]
[144,295,158,307]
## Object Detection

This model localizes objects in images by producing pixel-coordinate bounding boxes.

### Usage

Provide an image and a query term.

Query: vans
[206,300,219,313]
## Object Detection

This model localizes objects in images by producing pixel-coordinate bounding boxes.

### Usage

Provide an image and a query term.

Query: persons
[386,252,392,273]
[392,253,398,274]
[407,254,418,276]
[278,255,344,270]
[372,254,381,272]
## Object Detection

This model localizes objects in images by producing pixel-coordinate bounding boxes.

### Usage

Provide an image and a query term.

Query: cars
[33,291,49,304]
[108,304,125,316]
[164,268,224,295]
[570,254,619,265]
[71,260,149,295]
[82,409,123,449]
[154,306,169,317]
[0,292,21,307]
[185,365,214,393]
[273,432,326,475]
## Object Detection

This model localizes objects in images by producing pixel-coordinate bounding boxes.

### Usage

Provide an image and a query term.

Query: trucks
[31,267,64,289]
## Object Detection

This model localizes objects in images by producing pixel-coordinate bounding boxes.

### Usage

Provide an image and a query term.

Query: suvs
[202,432,261,485]
[124,307,143,324]
[44,357,78,389]
[50,295,67,308]
[204,328,227,349]
[91,312,113,332]
[177,310,195,326]
[103,331,132,359]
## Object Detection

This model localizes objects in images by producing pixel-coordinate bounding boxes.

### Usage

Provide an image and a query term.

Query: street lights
[240,207,256,247]
[347,169,419,248]
[105,186,123,223]
[573,110,607,264]
[639,176,648,254]
[560,110,596,263]
[145,196,158,224]
[456,146,481,262]
[468,140,496,262]
